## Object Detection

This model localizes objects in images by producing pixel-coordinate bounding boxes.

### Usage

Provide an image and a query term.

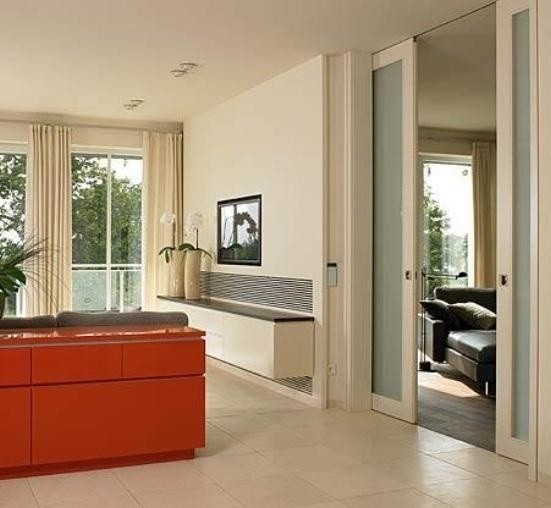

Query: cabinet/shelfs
[0,324,204,480]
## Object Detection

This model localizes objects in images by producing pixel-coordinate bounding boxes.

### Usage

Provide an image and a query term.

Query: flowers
[175,211,213,259]
[158,207,179,261]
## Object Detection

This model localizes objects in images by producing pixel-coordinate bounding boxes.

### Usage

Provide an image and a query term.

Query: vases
[167,249,183,297]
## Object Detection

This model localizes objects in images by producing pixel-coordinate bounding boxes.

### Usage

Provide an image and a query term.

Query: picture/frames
[182,249,201,299]
[215,193,261,268]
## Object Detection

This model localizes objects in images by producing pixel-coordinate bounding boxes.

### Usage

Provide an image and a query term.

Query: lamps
[420,267,467,371]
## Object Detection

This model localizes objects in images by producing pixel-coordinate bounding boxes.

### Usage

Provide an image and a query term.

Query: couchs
[414,285,495,395]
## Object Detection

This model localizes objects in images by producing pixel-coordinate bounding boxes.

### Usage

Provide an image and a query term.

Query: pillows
[417,297,463,328]
[448,300,495,329]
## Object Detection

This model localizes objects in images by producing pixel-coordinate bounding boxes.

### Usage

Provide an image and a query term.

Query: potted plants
[0,226,71,325]
